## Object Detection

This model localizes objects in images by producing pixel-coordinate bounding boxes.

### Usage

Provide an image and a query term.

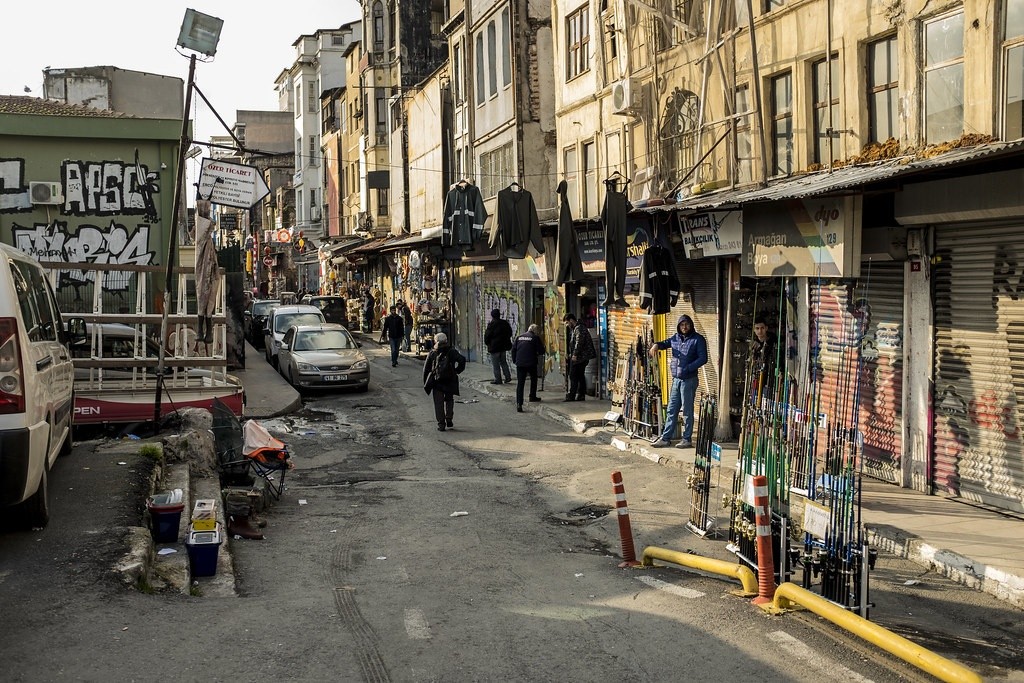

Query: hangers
[459,173,466,184]
[602,165,632,184]
[510,176,520,188]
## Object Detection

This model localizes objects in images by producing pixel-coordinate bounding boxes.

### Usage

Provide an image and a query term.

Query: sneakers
[674,437,693,448]
[650,436,671,447]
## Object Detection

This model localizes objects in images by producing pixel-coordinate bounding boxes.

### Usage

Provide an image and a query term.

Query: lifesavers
[408,250,421,269]
[277,229,290,243]
[421,276,436,292]
[418,298,431,315]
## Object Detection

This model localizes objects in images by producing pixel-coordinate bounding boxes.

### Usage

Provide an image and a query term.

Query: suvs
[243,297,281,347]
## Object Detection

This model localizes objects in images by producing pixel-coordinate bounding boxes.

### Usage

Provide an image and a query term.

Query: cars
[64,323,247,406]
[302,296,349,331]
[277,323,371,391]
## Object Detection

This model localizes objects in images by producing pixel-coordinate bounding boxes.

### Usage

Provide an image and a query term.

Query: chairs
[245,445,291,500]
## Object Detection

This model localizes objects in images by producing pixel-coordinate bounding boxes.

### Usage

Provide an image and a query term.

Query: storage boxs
[192,500,216,530]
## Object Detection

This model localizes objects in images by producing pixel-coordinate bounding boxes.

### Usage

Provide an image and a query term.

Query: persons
[296,287,319,302]
[484,309,513,384]
[751,317,778,346]
[422,333,466,431]
[649,314,708,448]
[563,313,596,402]
[396,299,413,352]
[511,324,545,412]
[364,290,375,334]
[260,278,276,300]
[381,306,404,367]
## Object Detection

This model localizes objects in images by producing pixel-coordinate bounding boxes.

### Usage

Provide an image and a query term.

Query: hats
[363,289,371,297]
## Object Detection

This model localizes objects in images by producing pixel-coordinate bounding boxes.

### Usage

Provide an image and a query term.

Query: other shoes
[563,397,575,402]
[437,427,446,431]
[489,381,501,384]
[447,422,454,427]
[575,396,585,401]
[516,403,523,412]
[529,395,542,402]
[504,378,511,383]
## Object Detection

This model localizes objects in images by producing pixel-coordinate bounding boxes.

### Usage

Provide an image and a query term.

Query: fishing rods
[621,321,663,442]
[682,218,881,620]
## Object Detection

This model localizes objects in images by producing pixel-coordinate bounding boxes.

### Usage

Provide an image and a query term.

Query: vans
[261,305,327,368]
[0,241,88,526]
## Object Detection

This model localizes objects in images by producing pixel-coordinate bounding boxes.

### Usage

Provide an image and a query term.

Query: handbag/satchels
[408,249,422,271]
[423,370,435,396]
[402,337,408,352]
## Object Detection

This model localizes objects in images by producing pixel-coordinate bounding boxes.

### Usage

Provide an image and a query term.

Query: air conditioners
[311,207,320,220]
[630,166,658,201]
[29,181,62,205]
[612,77,644,116]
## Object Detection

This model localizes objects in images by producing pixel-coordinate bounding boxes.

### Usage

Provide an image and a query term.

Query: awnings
[317,226,443,260]
[539,137,1024,227]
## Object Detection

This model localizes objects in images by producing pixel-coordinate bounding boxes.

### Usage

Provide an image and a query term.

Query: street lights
[154,8,224,434]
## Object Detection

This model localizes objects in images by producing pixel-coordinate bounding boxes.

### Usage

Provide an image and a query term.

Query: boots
[225,494,267,539]
